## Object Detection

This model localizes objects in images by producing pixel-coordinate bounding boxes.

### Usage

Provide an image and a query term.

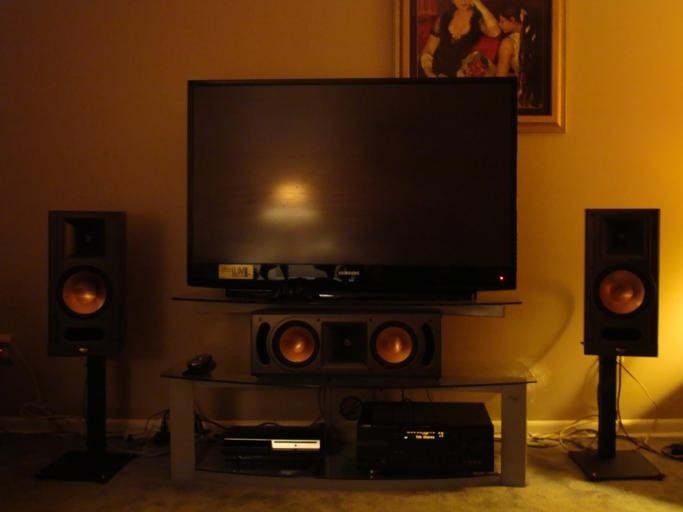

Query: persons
[419,0,501,78]
[493,7,539,108]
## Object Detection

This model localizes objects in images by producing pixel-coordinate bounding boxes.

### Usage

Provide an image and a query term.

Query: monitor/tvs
[187,77,517,301]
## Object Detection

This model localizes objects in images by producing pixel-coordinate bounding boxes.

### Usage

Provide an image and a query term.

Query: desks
[159,361,536,493]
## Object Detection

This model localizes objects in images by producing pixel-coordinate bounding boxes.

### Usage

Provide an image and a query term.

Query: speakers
[584,208,660,357]
[251,308,441,380]
[47,211,126,357]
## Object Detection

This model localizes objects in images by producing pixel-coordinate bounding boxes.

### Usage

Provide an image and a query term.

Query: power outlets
[0,333,16,367]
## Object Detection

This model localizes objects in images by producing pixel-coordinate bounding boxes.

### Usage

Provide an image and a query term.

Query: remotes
[188,353,212,368]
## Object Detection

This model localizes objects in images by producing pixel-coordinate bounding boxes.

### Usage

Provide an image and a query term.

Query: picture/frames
[390,0,567,136]
